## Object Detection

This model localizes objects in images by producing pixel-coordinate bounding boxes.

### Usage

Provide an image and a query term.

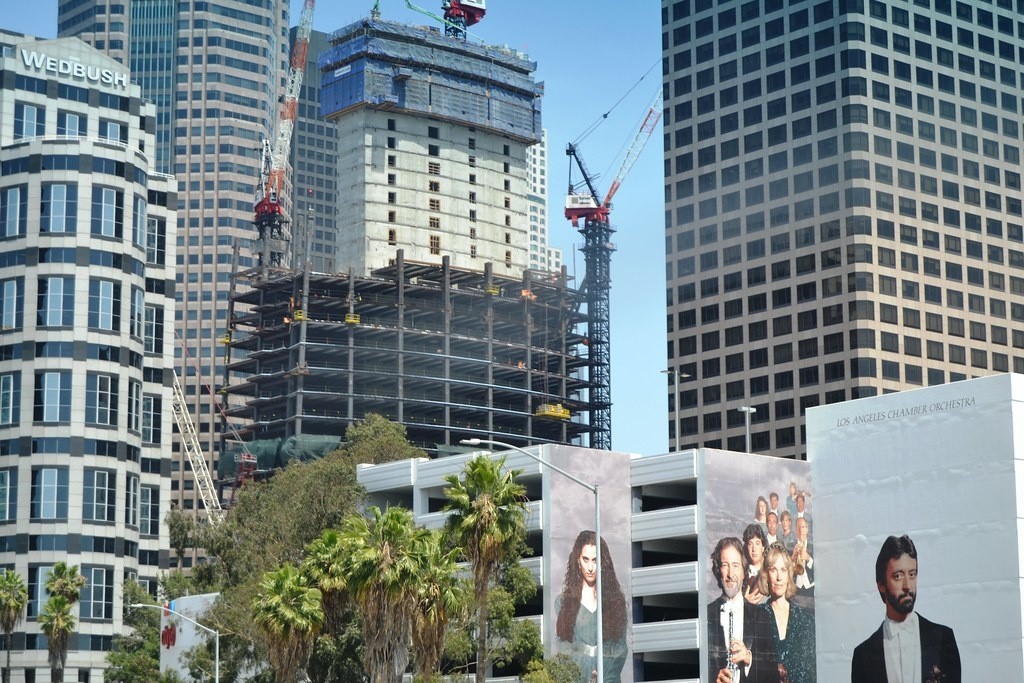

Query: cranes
[564,58,664,452]
[441,0,487,39]
[174,329,258,526]
[254,0,316,268]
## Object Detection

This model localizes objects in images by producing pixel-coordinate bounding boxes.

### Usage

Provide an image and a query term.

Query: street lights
[459,438,604,683]
[130,603,219,683]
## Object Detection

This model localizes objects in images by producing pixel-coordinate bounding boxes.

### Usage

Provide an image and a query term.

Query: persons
[850,533,962,683]
[705,482,815,683]
[552,529,628,682]
